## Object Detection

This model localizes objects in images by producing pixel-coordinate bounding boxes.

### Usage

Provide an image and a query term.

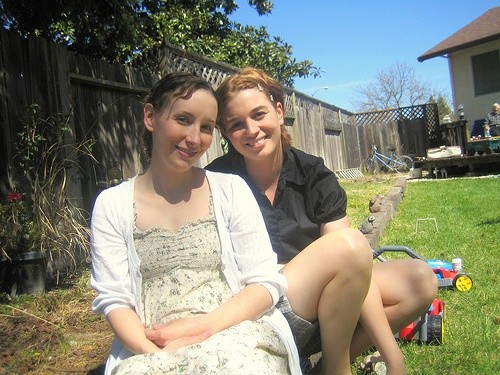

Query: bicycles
[361,144,414,176]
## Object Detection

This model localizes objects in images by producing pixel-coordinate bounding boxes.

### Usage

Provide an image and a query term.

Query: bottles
[458,109,464,120]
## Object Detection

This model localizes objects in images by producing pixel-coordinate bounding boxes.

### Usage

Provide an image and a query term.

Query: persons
[90,73,303,375]
[482,103,499,137]
[204,67,438,375]
